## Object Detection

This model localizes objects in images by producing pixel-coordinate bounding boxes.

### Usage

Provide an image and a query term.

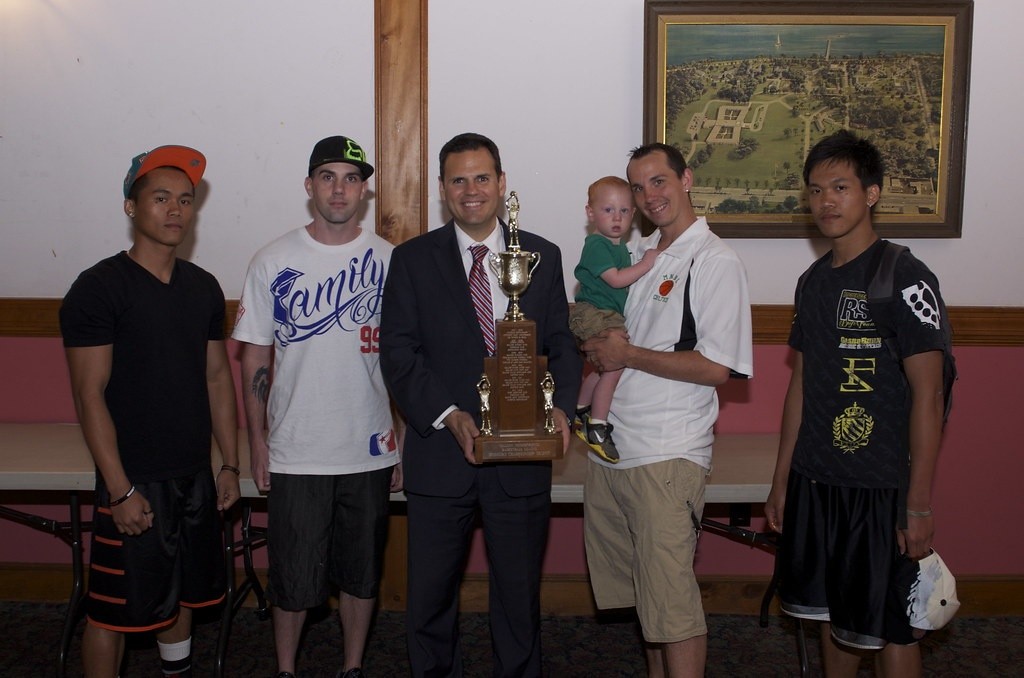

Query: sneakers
[573,404,591,432]
[575,413,620,463]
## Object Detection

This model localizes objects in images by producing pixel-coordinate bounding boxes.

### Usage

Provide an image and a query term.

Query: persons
[581,143,756,678]
[541,369,557,429]
[58,146,240,678]
[229,136,406,678]
[476,373,493,436]
[378,133,584,678]
[221,465,240,476]
[764,129,952,678]
[505,191,520,245]
[568,177,662,464]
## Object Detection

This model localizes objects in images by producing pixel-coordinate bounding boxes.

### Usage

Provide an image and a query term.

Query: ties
[467,244,496,359]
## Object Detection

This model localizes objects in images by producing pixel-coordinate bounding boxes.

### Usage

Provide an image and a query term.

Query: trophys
[474,191,564,461]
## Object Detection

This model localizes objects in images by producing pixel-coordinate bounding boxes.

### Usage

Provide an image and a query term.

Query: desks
[0,421,811,678]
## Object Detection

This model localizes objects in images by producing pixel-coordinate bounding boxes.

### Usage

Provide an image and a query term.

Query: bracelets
[109,486,136,506]
[907,505,934,517]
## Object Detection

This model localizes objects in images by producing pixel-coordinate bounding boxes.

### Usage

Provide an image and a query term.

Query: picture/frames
[643,0,975,240]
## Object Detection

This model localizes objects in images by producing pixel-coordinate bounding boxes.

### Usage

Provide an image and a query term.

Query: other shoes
[337,666,367,678]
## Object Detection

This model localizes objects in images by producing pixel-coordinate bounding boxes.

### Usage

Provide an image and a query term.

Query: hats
[124,144,207,199]
[308,136,374,182]
[881,543,962,645]
